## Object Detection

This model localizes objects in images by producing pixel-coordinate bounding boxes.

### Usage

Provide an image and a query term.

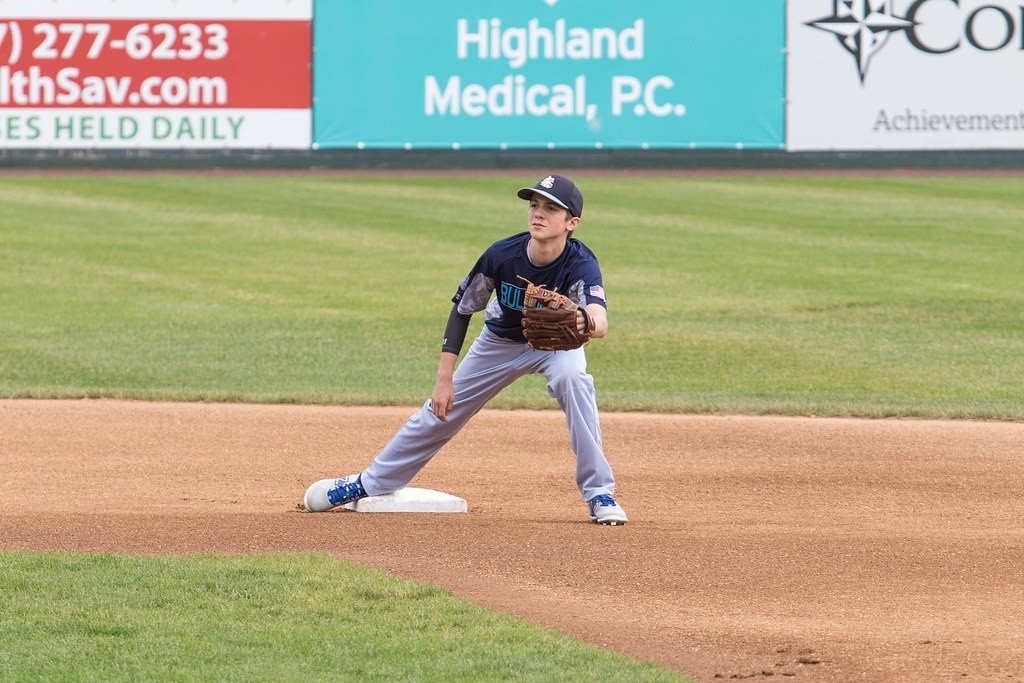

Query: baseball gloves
[516,273,596,355]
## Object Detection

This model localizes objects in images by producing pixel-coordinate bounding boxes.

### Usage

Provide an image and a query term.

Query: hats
[516,175,583,218]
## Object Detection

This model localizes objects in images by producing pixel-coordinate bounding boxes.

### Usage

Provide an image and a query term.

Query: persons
[303,175,628,525]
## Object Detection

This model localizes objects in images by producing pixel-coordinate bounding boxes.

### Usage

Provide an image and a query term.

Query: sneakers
[588,494,628,526]
[303,474,369,513]
